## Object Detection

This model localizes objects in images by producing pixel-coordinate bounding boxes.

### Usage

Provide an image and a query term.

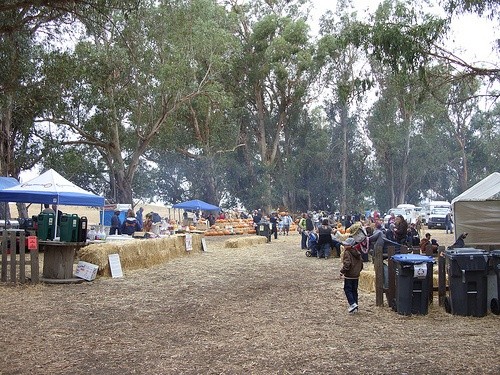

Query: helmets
[425,233,431,237]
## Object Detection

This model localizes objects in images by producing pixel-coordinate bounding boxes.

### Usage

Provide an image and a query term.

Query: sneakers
[348,303,359,314]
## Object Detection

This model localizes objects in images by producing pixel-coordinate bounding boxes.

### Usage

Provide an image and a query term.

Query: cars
[384,208,412,229]
[397,201,450,225]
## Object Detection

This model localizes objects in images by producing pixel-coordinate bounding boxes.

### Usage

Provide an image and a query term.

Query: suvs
[427,207,453,229]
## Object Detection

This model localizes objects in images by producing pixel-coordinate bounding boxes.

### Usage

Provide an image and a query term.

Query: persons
[43,202,63,238]
[233,209,454,262]
[339,238,364,312]
[111,206,229,235]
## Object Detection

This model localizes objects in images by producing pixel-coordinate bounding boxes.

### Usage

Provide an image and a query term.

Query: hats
[342,238,356,245]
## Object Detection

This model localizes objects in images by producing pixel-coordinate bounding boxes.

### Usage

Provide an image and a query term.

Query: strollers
[303,229,325,257]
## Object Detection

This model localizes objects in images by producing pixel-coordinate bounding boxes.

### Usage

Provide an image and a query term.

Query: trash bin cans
[34,211,89,244]
[391,254,432,318]
[443,245,500,321]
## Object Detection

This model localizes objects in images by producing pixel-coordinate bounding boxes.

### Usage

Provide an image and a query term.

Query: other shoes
[318,257,319,259]
[325,256,328,260]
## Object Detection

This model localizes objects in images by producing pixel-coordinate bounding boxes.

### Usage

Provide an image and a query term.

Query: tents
[172,199,221,219]
[0,168,105,240]
